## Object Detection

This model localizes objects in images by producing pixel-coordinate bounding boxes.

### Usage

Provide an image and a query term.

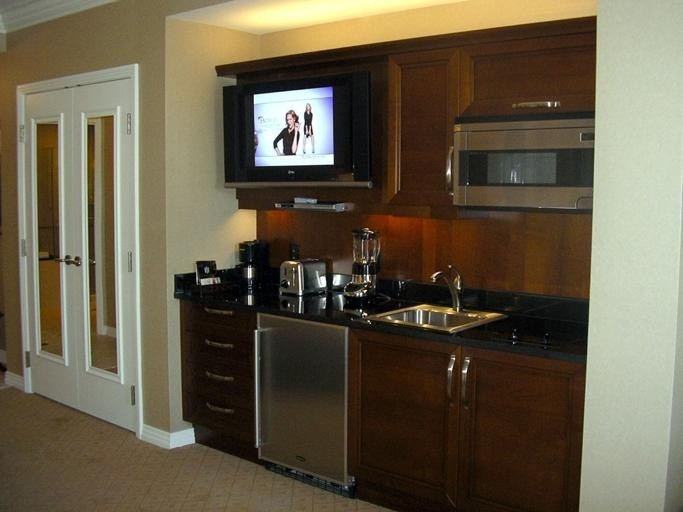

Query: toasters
[279,258,328,297]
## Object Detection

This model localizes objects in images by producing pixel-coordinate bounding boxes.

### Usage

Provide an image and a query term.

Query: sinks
[363,302,510,335]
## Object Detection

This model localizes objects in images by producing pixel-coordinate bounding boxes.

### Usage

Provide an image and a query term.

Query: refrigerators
[255,313,348,489]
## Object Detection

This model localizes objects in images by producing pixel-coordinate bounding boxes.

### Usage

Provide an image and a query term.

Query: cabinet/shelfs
[349,331,588,512]
[386,54,458,205]
[180,300,349,493]
[463,46,592,112]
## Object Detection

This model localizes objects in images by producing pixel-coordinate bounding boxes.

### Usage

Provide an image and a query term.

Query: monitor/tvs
[219,69,376,190]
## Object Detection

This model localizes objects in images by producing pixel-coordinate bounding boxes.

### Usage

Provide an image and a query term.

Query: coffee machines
[352,228,381,298]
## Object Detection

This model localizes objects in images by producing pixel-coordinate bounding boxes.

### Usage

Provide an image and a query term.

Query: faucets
[430,264,464,313]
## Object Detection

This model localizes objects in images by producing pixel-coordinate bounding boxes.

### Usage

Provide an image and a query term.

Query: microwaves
[454,111,595,213]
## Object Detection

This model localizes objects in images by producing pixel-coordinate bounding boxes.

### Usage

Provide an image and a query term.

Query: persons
[272,109,302,157]
[302,103,314,154]
